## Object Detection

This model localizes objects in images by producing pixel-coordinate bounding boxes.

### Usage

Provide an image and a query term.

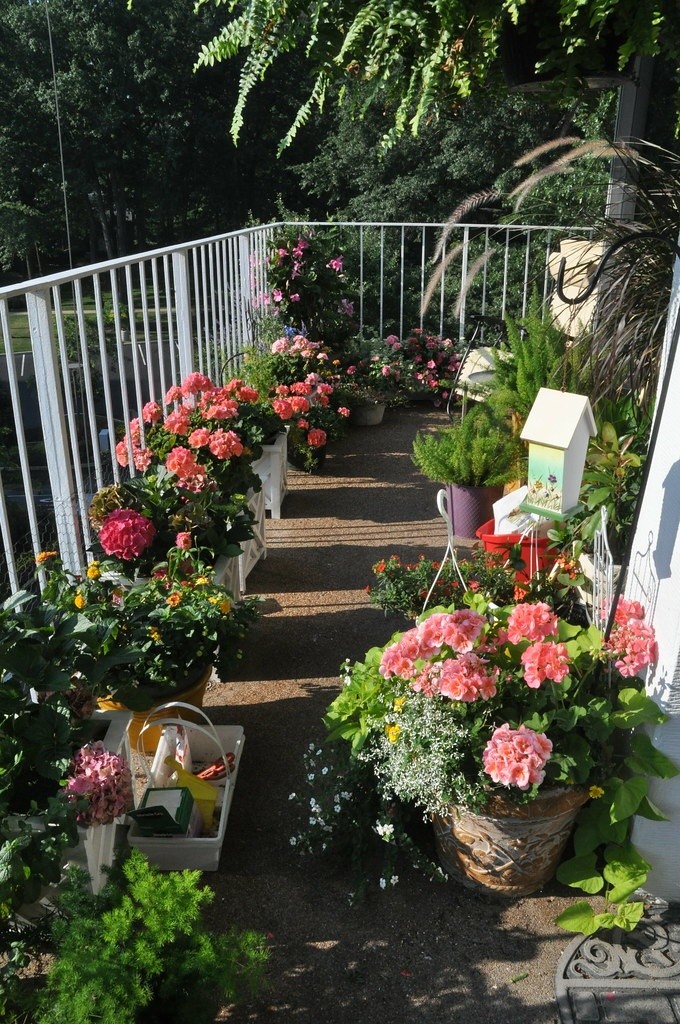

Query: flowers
[32,239,658,894]
